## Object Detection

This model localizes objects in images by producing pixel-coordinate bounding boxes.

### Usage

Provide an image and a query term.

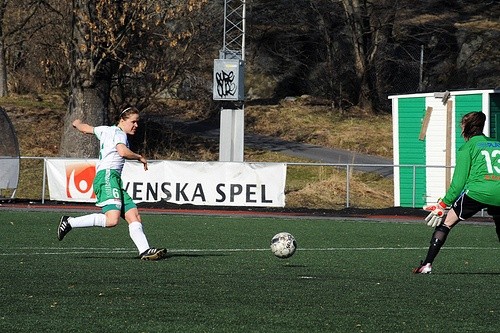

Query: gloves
[423,201,447,227]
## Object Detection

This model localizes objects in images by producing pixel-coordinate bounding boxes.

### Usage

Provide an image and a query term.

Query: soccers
[269,231,297,259]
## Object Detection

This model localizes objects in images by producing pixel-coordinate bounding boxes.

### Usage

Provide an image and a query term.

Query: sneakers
[140,248,167,260]
[57,216,72,241]
[412,263,432,274]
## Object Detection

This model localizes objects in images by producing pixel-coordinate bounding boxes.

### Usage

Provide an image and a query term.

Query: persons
[411,112,500,274]
[57,106,167,261]
